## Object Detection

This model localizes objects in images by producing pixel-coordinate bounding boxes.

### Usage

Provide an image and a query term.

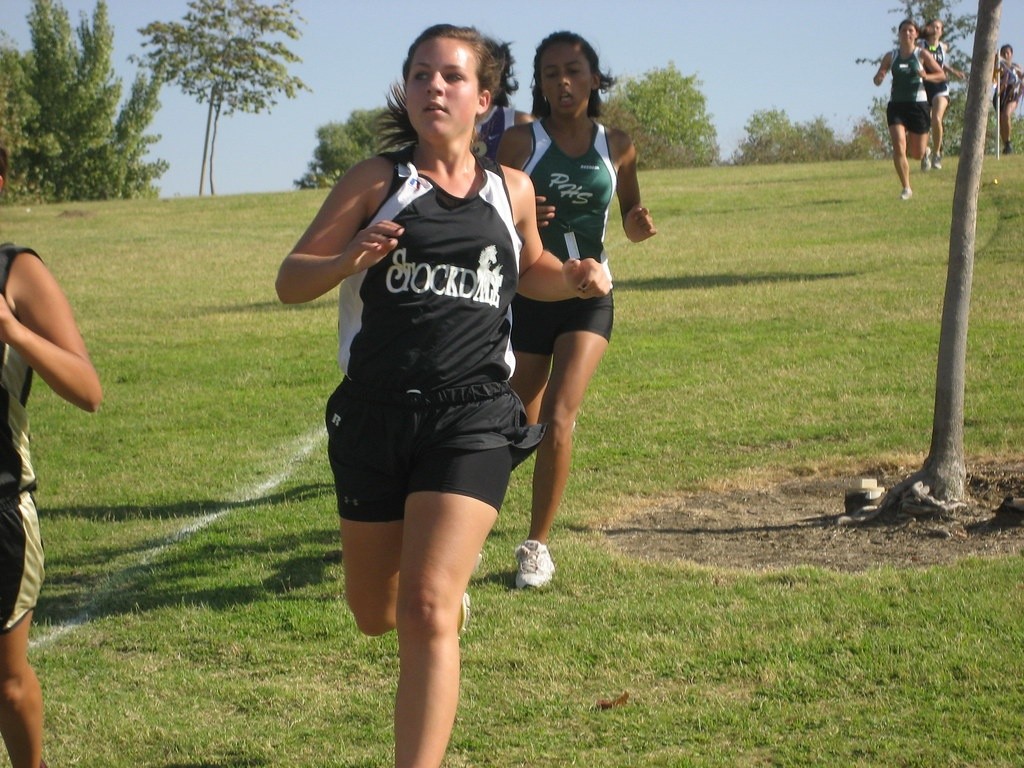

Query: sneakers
[515,541,555,591]
[921,147,932,172]
[932,153,941,170]
[898,189,912,199]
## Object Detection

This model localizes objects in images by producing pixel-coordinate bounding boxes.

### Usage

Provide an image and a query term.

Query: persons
[872,18,965,201]
[276,21,656,768]
[991,44,1024,156]
[0,146,102,768]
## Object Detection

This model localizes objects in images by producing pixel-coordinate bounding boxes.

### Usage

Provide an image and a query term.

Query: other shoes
[457,592,470,641]
[1003,144,1011,154]
